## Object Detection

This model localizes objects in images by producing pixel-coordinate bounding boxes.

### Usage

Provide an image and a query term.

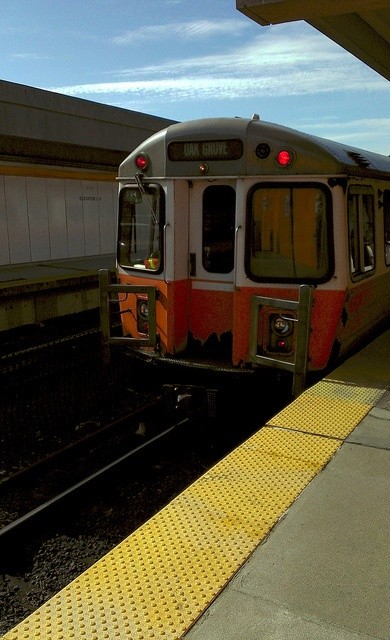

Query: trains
[98,114,390,402]
